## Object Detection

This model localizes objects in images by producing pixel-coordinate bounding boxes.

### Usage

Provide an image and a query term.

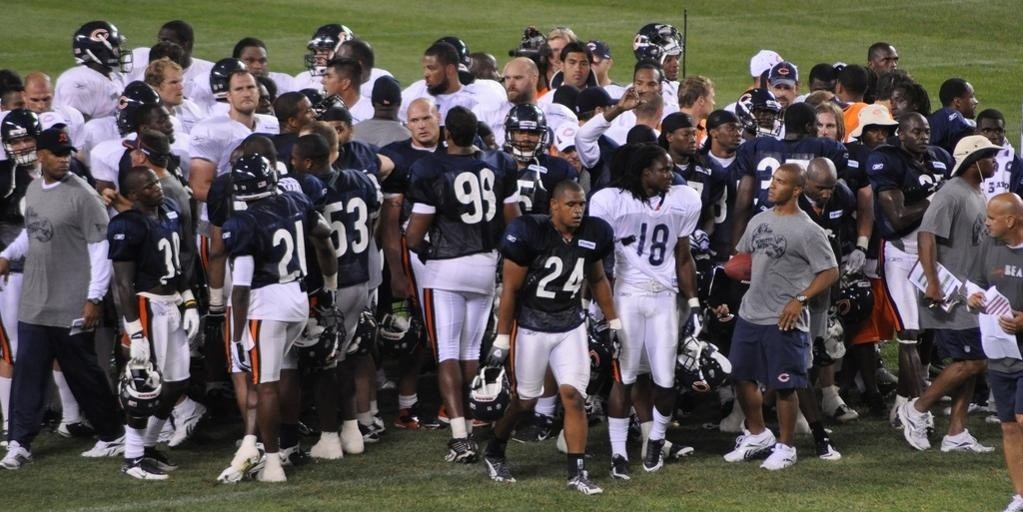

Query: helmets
[1,108,42,168]
[118,81,161,131]
[73,21,133,73]
[839,273,875,328]
[293,308,346,370]
[632,23,682,65]
[675,337,732,393]
[117,360,162,419]
[736,88,785,137]
[503,104,547,162]
[210,59,246,102]
[813,306,846,367]
[347,312,379,360]
[436,36,473,74]
[230,153,277,201]
[304,24,354,77]
[469,366,510,421]
[379,314,426,360]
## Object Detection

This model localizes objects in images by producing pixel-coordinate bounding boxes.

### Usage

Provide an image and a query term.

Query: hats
[751,50,783,78]
[950,135,1005,177]
[554,122,580,152]
[372,76,401,106]
[706,109,738,130]
[122,132,170,160]
[37,128,78,153]
[586,40,611,59]
[768,62,798,88]
[661,112,693,133]
[575,87,619,114]
[852,105,900,138]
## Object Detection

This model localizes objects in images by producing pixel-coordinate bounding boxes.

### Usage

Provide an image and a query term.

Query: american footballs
[725,252,752,279]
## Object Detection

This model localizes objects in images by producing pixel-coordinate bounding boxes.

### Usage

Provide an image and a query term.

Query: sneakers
[358,421,381,443]
[340,430,364,454]
[724,428,776,462]
[218,447,260,484]
[608,454,631,481]
[311,444,343,459]
[394,408,440,431]
[816,438,841,461]
[512,423,551,444]
[484,454,516,483]
[643,439,665,473]
[81,433,126,458]
[257,466,288,482]
[168,402,206,447]
[822,399,859,420]
[145,451,179,472]
[941,429,995,454]
[156,423,175,443]
[0,440,34,470]
[438,405,489,427]
[58,417,97,439]
[279,448,299,466]
[373,412,385,433]
[641,440,694,461]
[567,471,602,495]
[899,398,931,451]
[121,458,168,480]
[760,443,796,471]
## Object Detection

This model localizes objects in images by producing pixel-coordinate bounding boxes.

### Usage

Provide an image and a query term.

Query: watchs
[88,298,103,306]
[796,294,808,308]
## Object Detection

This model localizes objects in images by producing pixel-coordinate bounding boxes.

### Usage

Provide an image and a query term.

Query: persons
[481,179,628,497]
[895,133,1006,457]
[403,106,523,464]
[588,145,705,482]
[0,129,126,471]
[1,20,1023,484]
[722,163,841,471]
[964,192,1023,512]
[106,165,202,482]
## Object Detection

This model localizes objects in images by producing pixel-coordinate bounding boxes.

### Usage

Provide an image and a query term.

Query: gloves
[608,318,623,359]
[130,331,150,366]
[184,300,199,339]
[683,297,704,337]
[485,334,510,366]
[232,340,252,375]
[204,302,226,341]
[314,289,337,312]
[845,246,867,275]
